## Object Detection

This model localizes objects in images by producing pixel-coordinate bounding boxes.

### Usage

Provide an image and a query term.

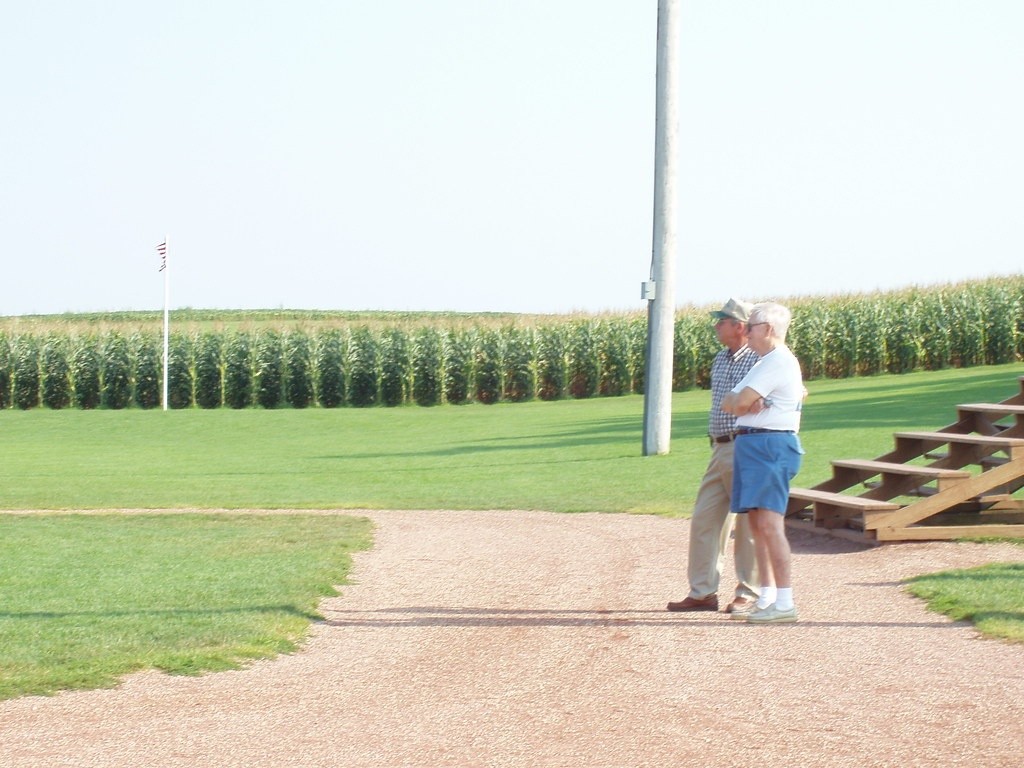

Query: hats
[709,297,753,322]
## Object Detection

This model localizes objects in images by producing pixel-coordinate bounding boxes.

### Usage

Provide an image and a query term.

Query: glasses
[747,322,768,332]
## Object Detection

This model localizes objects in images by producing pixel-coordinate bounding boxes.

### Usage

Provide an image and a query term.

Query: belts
[736,429,795,434]
[716,434,736,443]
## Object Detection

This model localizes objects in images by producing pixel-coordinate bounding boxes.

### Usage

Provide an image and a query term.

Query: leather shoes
[667,593,718,611]
[726,596,755,613]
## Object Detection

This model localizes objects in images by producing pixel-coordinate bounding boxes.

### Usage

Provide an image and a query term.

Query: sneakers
[746,603,797,624]
[730,601,765,619]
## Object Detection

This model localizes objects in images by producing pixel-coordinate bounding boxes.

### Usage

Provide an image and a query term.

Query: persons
[667,298,809,613]
[721,302,807,623]
